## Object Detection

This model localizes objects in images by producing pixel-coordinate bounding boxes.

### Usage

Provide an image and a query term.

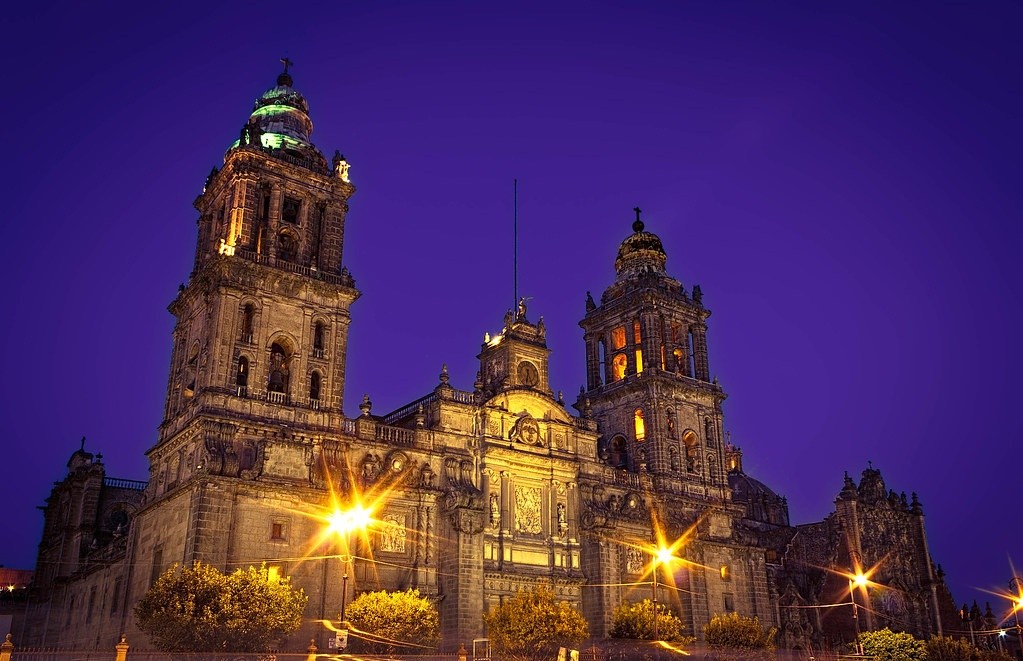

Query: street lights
[328,508,366,653]
[1012,595,1023,657]
[653,549,671,643]
[847,562,867,661]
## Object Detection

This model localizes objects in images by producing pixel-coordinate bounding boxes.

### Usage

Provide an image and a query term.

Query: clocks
[517,361,539,388]
